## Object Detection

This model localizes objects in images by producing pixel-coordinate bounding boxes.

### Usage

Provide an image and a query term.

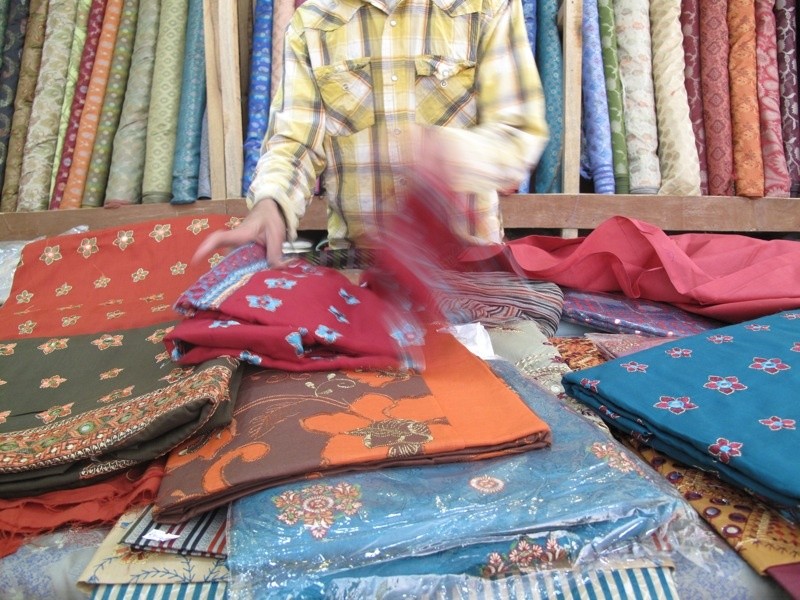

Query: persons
[189,0,550,266]
